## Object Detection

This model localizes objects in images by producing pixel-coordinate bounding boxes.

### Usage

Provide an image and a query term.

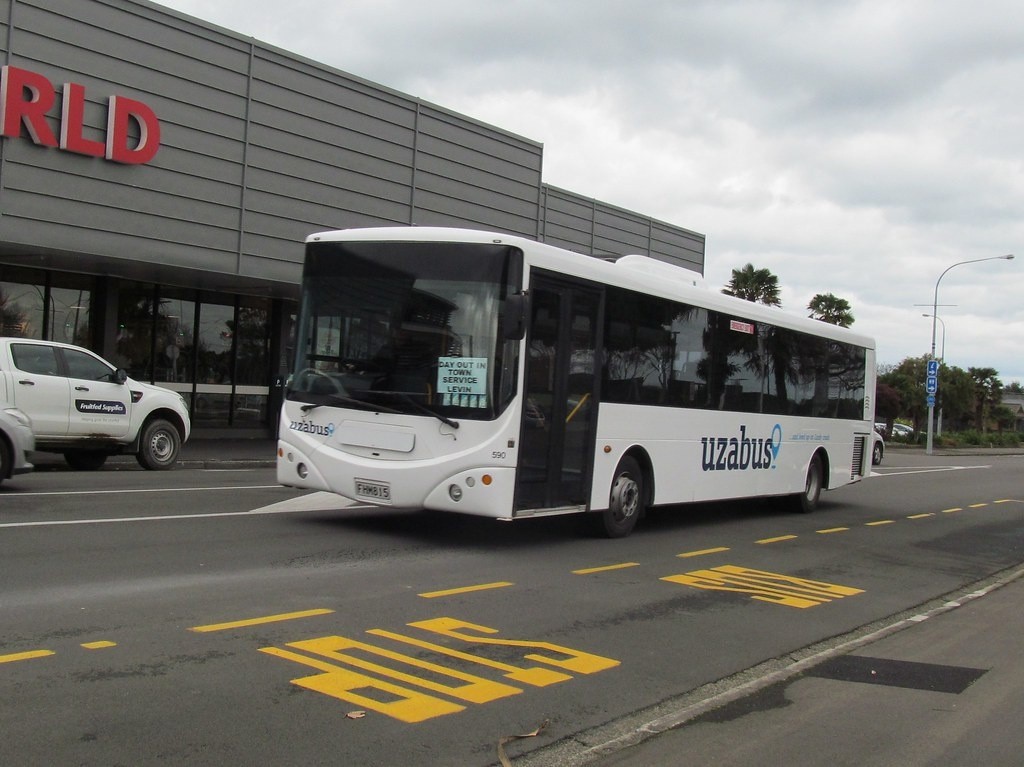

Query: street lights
[922,312,947,439]
[924,252,1015,458]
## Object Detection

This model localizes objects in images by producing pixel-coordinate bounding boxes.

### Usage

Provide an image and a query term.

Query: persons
[347,320,434,376]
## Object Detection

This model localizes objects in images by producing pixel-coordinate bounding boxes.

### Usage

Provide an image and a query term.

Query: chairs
[197,397,216,420]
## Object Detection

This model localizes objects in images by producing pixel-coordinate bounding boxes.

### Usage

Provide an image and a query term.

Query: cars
[875,422,918,437]
[0,398,36,488]
[0,335,191,472]
[874,428,884,465]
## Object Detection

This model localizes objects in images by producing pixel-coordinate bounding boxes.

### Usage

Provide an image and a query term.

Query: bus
[274,223,878,537]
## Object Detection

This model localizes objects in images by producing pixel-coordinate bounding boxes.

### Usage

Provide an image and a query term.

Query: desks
[214,401,244,421]
